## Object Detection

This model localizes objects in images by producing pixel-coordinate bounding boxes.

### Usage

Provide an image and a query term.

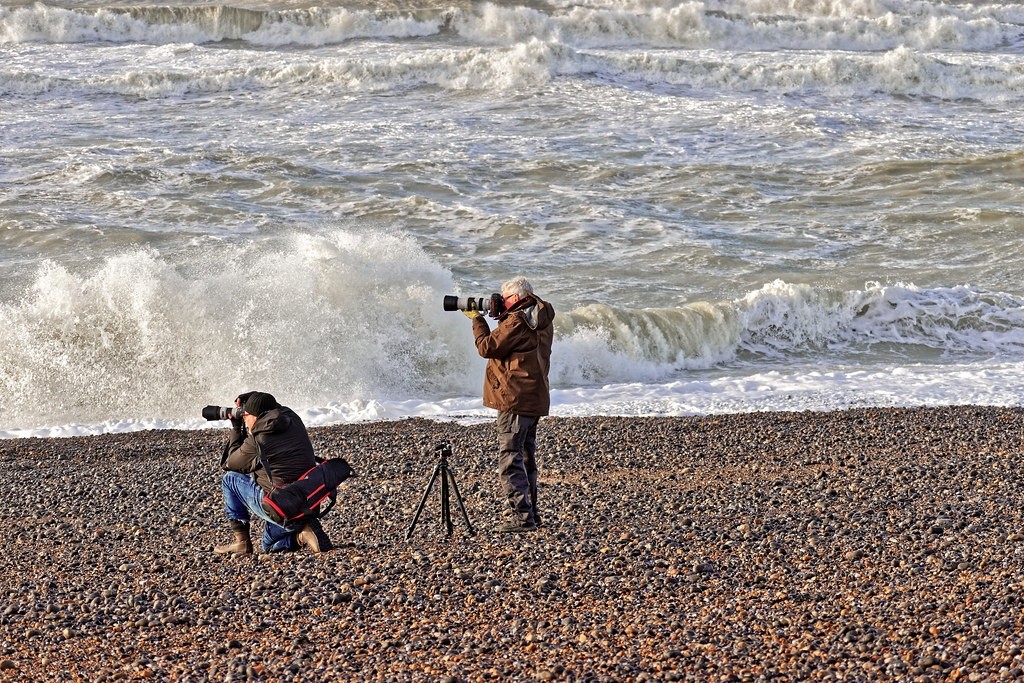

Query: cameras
[202,405,248,421]
[444,294,504,317]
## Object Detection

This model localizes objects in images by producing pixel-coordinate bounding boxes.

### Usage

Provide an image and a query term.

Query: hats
[243,392,276,418]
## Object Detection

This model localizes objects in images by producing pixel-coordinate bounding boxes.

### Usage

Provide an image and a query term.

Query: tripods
[405,441,475,538]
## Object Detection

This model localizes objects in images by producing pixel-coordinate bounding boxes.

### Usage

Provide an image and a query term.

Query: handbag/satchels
[262,455,358,528]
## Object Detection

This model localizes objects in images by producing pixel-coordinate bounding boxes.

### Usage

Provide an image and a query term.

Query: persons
[460,277,555,532]
[214,391,333,554]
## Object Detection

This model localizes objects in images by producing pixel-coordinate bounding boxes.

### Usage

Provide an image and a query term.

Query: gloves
[461,301,481,319]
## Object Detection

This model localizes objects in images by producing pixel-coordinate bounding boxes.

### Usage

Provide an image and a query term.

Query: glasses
[499,293,515,303]
[241,412,251,420]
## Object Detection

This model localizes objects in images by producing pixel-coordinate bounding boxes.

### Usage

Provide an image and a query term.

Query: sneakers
[500,522,538,531]
[532,515,543,527]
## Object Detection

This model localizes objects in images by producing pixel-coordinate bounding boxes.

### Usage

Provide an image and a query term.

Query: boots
[214,530,253,555]
[298,521,334,553]
